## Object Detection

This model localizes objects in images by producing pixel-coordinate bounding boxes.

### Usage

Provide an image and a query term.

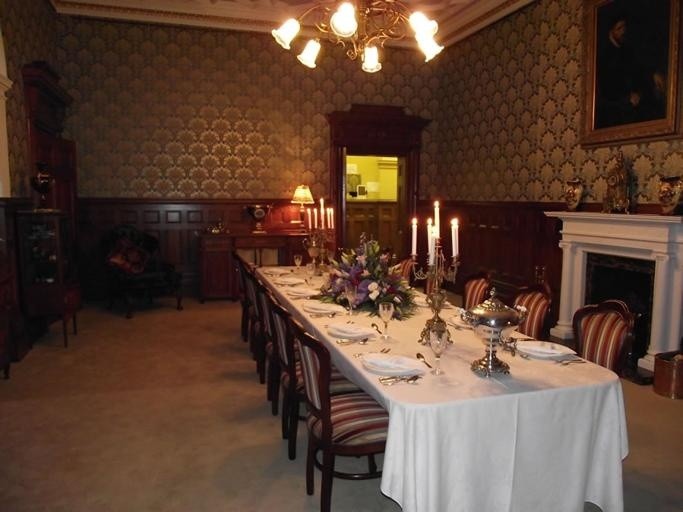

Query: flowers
[311,232,416,323]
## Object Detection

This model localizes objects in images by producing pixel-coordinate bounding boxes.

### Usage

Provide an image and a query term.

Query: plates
[448,316,472,330]
[518,341,567,357]
[366,355,418,373]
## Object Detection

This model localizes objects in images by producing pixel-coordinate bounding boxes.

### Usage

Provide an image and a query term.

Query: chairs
[288,315,388,512]
[231,250,298,441]
[570,298,636,377]
[97,223,186,320]
[461,272,493,312]
[505,281,553,341]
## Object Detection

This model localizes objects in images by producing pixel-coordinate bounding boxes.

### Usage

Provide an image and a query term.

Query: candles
[410,200,460,265]
[307,195,335,230]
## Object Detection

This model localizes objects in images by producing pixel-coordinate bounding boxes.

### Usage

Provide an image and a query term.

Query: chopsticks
[415,351,432,370]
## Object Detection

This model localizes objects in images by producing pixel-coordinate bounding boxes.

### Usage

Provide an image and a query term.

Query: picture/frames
[578,0,682,151]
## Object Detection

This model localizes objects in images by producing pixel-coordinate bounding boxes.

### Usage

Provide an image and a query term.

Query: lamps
[269,0,446,75]
[290,184,314,229]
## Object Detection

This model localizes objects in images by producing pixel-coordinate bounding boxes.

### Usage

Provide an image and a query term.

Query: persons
[593,16,665,128]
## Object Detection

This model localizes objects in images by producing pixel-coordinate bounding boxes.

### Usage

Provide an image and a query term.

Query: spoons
[382,375,418,385]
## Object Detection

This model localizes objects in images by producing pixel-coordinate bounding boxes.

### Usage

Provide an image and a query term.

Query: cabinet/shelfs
[195,230,312,305]
[15,208,84,349]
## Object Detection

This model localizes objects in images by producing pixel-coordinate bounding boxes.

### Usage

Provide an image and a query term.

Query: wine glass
[263,255,395,345]
[430,328,446,375]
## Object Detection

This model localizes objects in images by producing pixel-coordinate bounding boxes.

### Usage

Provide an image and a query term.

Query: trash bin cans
[653,351,683,400]
